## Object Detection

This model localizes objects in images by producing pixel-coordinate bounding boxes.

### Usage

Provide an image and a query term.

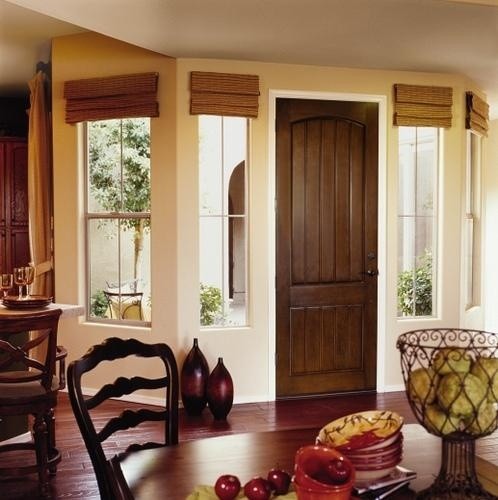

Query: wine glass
[23,266,36,300]
[13,268,29,301]
[1,274,14,298]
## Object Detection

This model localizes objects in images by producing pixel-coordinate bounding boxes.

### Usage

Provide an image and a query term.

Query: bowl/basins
[293,446,357,500]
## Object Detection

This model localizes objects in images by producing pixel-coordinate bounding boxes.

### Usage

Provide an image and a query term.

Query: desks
[0,300,85,481]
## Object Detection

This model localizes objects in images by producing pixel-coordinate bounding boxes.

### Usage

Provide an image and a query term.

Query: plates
[2,295,53,310]
[316,408,405,482]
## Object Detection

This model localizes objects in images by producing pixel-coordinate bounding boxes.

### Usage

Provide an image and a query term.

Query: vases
[180,337,210,416]
[206,358,234,420]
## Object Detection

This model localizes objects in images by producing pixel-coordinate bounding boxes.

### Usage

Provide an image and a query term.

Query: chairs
[68,337,179,500]
[2,308,66,500]
[103,291,146,321]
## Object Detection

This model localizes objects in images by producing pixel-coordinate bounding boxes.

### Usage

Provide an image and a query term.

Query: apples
[471,357,498,403]
[243,477,272,500]
[432,346,469,373]
[267,467,291,494]
[462,403,495,433]
[213,474,241,500]
[436,374,488,419]
[406,368,439,404]
[425,404,459,435]
